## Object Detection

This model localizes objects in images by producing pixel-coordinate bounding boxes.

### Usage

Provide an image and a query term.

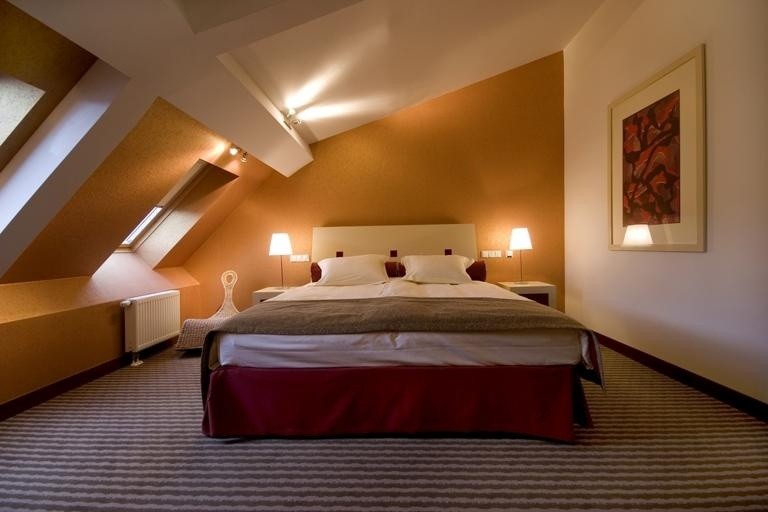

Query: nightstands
[496,280,556,310]
[252,286,297,306]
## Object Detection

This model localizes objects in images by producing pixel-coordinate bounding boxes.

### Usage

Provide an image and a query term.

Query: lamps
[509,227,533,284]
[268,233,293,290]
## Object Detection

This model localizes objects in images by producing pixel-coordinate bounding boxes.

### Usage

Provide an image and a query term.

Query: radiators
[120,289,181,366]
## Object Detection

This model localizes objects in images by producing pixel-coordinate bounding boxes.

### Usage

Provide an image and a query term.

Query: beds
[200,223,608,439]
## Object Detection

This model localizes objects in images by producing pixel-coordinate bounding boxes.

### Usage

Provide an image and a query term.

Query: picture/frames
[607,44,708,253]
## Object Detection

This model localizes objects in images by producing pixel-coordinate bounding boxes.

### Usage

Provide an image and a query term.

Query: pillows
[310,253,487,286]
